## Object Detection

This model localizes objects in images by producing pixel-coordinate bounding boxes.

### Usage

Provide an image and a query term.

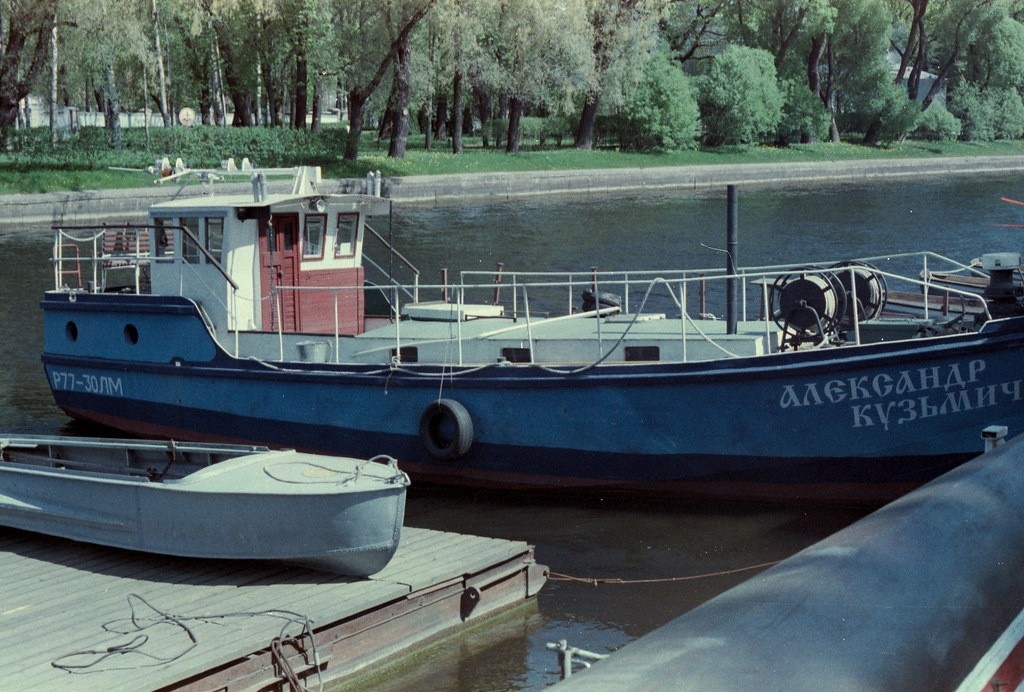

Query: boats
[0,435,410,577]
[35,163,1024,503]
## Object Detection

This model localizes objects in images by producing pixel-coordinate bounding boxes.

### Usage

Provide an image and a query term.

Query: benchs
[102,230,174,294]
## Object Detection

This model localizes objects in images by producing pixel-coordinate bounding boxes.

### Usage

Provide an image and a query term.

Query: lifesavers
[415,398,475,461]
